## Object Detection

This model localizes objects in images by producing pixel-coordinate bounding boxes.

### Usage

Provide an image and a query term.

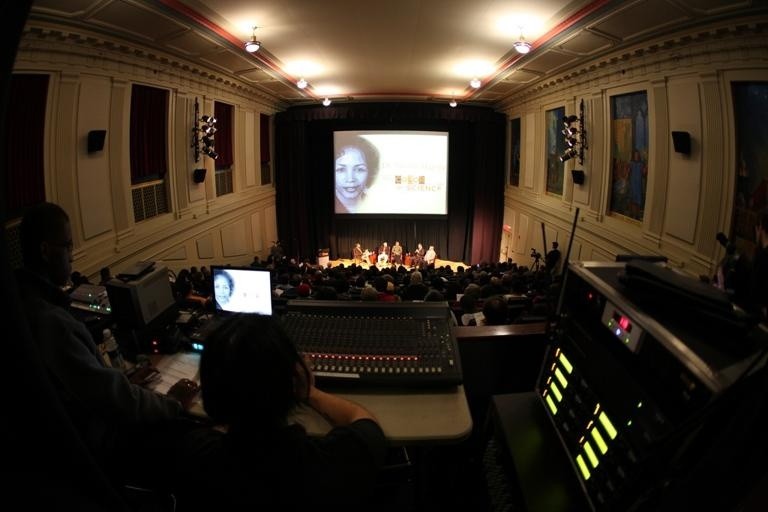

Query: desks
[404,255,411,267]
[369,254,377,264]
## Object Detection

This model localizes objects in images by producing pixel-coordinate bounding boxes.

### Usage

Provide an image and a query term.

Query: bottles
[102,328,126,370]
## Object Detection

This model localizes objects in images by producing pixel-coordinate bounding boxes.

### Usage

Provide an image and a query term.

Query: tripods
[530,259,540,271]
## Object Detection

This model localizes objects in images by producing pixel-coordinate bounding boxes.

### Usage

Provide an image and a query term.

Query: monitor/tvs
[205,264,277,321]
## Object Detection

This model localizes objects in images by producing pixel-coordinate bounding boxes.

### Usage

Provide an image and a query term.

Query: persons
[15,204,201,421]
[214,270,234,312]
[152,313,385,512]
[750,209,768,321]
[250,242,564,326]
[715,232,736,290]
[64,266,211,326]
[334,135,379,214]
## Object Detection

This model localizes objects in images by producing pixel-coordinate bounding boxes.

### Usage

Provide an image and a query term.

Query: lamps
[560,98,588,163]
[193,97,219,162]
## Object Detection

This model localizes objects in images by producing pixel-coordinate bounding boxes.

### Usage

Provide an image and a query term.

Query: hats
[298,283,311,298]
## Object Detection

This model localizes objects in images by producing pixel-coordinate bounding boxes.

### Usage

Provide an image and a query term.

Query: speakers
[192,168,207,183]
[571,169,585,185]
[672,131,691,153]
[88,130,107,154]
